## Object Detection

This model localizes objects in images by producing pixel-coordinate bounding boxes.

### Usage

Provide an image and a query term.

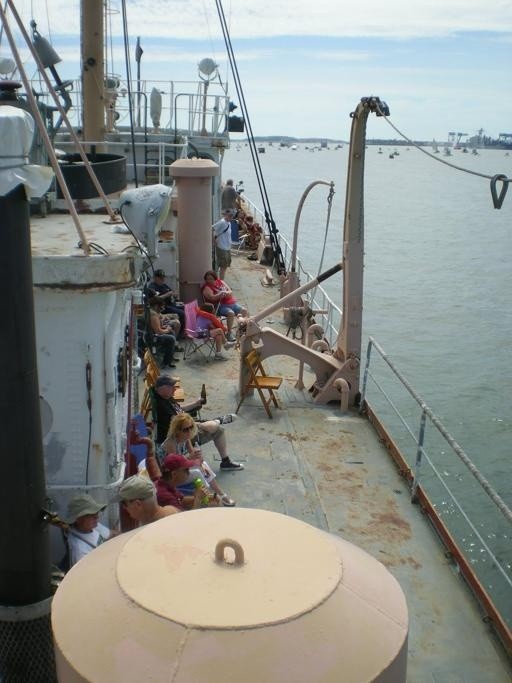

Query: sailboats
[430,139,452,157]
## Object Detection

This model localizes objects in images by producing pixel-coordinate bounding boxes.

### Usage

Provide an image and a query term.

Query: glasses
[183,425,193,432]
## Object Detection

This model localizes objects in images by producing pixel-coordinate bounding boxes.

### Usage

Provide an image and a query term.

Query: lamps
[119,184,172,264]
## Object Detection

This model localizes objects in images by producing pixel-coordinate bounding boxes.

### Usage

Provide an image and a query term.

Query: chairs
[230,219,246,255]
[141,291,282,460]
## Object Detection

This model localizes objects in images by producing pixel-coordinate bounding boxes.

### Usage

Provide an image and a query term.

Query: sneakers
[217,493,235,505]
[213,414,238,424]
[175,345,184,352]
[214,332,236,360]
[219,460,244,470]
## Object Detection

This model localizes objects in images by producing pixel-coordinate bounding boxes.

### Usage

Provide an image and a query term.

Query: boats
[235,138,411,160]
[462,147,510,157]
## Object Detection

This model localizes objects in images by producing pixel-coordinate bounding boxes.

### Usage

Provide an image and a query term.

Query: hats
[62,494,108,525]
[154,269,167,279]
[221,207,236,215]
[109,475,157,503]
[160,453,194,473]
[149,296,163,304]
[156,374,182,388]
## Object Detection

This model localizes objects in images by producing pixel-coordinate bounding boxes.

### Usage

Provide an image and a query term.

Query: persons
[154,411,237,507]
[151,381,210,421]
[109,474,182,526]
[150,373,244,470]
[152,452,211,513]
[66,492,119,572]
[144,176,264,371]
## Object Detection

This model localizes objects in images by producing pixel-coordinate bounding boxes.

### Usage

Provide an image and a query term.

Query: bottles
[201,383,207,406]
[170,290,173,305]
[194,442,202,469]
[191,473,213,505]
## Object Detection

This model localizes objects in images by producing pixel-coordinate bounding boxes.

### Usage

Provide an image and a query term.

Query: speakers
[227,115,245,132]
